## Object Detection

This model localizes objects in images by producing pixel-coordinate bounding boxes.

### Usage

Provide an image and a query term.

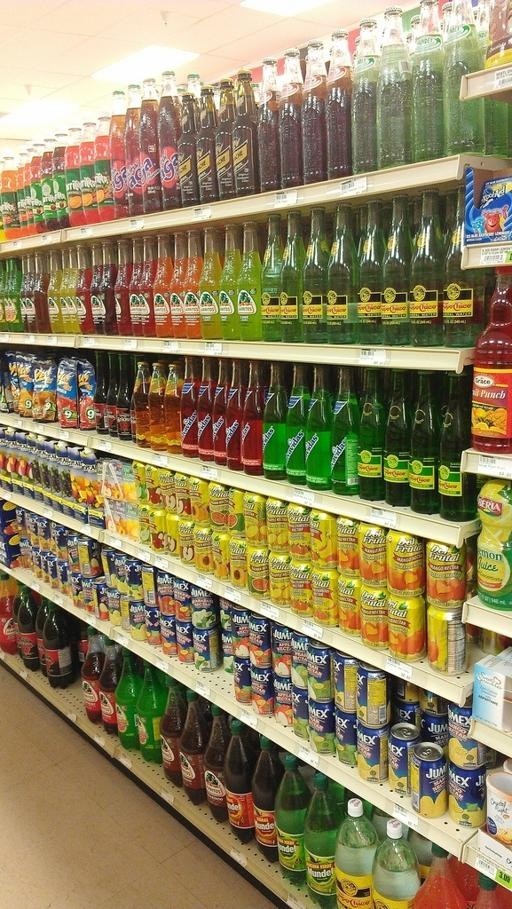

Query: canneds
[92,546,222,673]
[13,508,103,614]
[218,598,507,828]
[132,461,512,676]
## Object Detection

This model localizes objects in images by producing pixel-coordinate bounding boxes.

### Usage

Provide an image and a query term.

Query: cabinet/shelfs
[453,63,512,895]
[0,149,507,908]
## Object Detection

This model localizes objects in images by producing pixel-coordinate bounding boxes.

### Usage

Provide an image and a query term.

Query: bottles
[474,474,512,613]
[94,351,478,522]
[471,266,512,456]
[0,184,490,347]
[0,0,512,245]
[0,573,512,909]
